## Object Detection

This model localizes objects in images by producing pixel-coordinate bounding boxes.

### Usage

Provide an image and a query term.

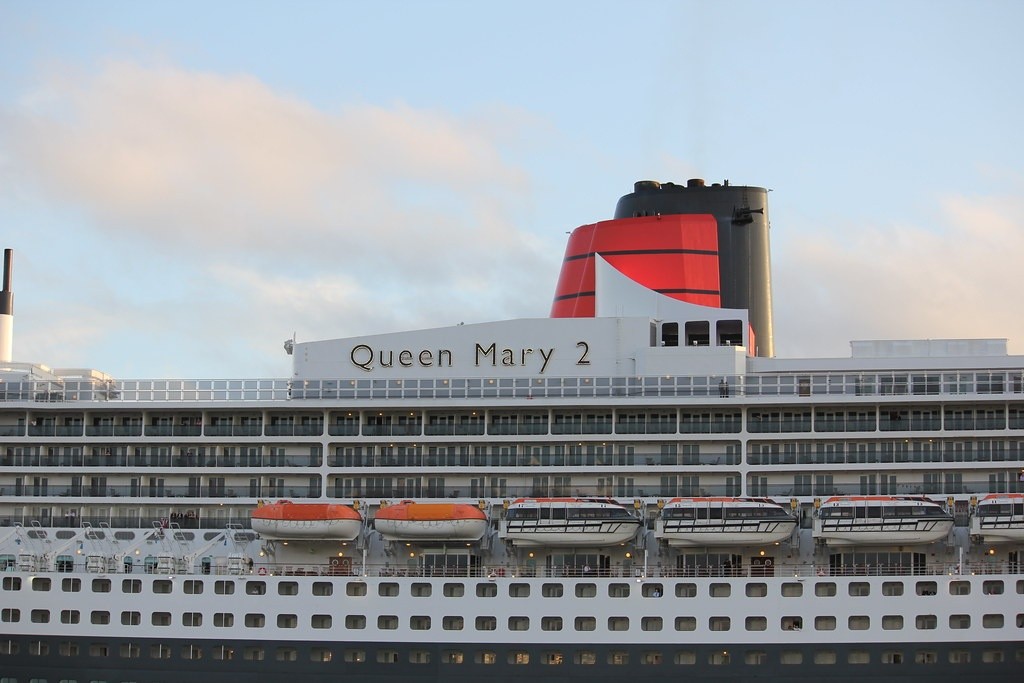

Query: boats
[372,499,490,540]
[249,498,361,544]
[656,498,801,550]
[499,496,641,550]
[967,492,1024,544]
[814,495,956,549]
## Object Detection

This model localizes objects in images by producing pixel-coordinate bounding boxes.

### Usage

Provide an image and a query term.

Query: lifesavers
[816,568,825,577]
[258,567,266,576]
[765,559,772,566]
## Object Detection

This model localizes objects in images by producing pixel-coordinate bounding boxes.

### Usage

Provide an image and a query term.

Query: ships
[0,179,1024,683]
